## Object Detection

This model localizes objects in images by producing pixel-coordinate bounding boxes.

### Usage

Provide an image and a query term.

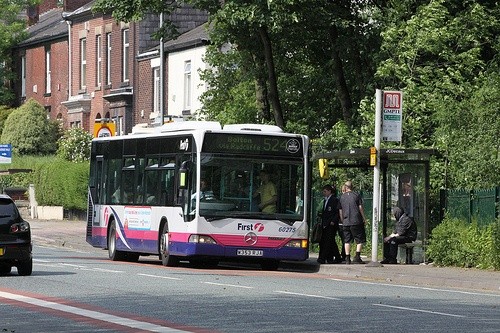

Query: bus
[84,121,330,266]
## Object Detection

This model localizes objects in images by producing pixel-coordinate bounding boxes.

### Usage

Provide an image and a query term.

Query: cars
[0,195,32,275]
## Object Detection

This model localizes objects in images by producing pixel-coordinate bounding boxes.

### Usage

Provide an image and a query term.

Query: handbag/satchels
[311,222,321,244]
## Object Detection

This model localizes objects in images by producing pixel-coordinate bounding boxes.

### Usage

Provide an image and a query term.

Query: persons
[110,128,284,217]
[381,206,418,265]
[339,180,367,263]
[311,184,342,264]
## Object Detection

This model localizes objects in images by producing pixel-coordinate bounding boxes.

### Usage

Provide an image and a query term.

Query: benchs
[396,243,420,264]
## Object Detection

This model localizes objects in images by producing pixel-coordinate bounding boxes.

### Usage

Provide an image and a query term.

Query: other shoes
[381,258,390,263]
[317,254,364,264]
[388,259,397,263]
